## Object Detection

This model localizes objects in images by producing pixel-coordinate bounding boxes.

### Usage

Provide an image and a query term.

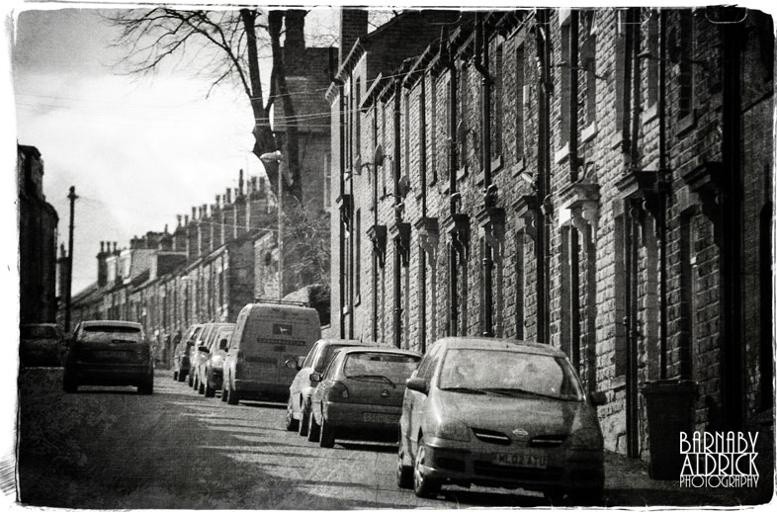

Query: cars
[284,338,423,448]
[20,319,153,394]
[174,321,236,398]
[397,336,608,501]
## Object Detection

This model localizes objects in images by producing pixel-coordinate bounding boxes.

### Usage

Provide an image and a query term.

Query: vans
[221,302,324,403]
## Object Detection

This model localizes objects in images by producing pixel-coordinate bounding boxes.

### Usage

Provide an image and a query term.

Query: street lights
[261,152,286,297]
